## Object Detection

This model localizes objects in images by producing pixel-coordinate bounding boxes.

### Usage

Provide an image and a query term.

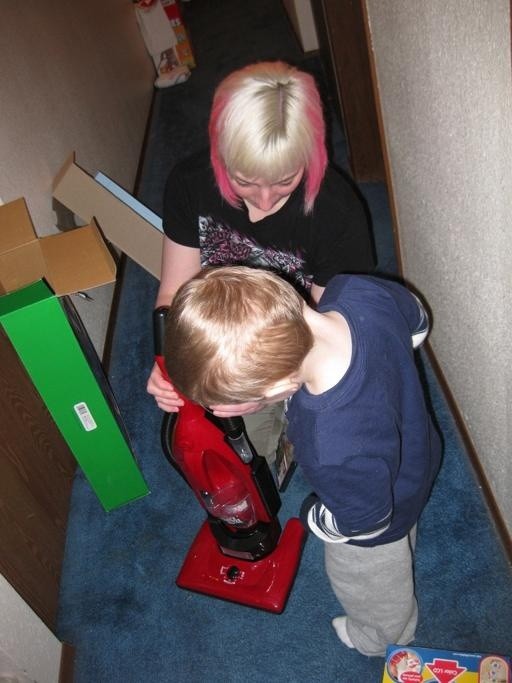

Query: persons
[146,60,378,484]
[165,264,445,656]
